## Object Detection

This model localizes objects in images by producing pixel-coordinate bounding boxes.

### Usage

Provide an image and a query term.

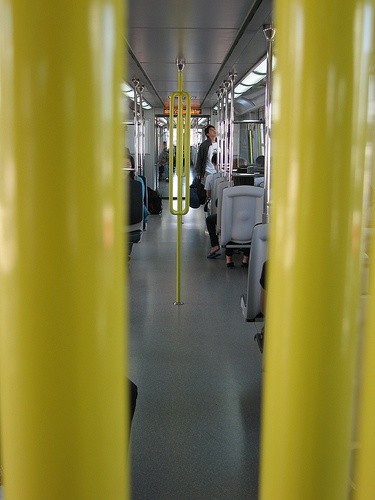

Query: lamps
[121,79,153,110]
[213,54,277,112]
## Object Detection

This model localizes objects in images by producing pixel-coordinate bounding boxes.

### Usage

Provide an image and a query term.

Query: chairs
[210,171,222,210]
[217,184,266,250]
[240,222,270,323]
[215,180,234,234]
[212,176,225,213]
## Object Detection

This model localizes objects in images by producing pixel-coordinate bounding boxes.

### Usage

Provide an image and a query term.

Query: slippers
[207,253,221,258]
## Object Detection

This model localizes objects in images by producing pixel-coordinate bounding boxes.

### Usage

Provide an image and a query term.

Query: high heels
[242,262,248,270]
[226,262,234,269]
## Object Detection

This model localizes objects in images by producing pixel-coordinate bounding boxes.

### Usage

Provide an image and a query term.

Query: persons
[259,261,268,338]
[225,168,252,268]
[204,152,222,258]
[159,140,196,183]
[231,154,265,187]
[188,124,218,203]
[126,150,143,265]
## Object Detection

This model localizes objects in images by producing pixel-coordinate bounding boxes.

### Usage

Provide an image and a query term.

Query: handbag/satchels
[190,178,205,208]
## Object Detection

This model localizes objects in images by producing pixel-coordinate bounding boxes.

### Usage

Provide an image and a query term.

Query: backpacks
[147,186,163,215]
